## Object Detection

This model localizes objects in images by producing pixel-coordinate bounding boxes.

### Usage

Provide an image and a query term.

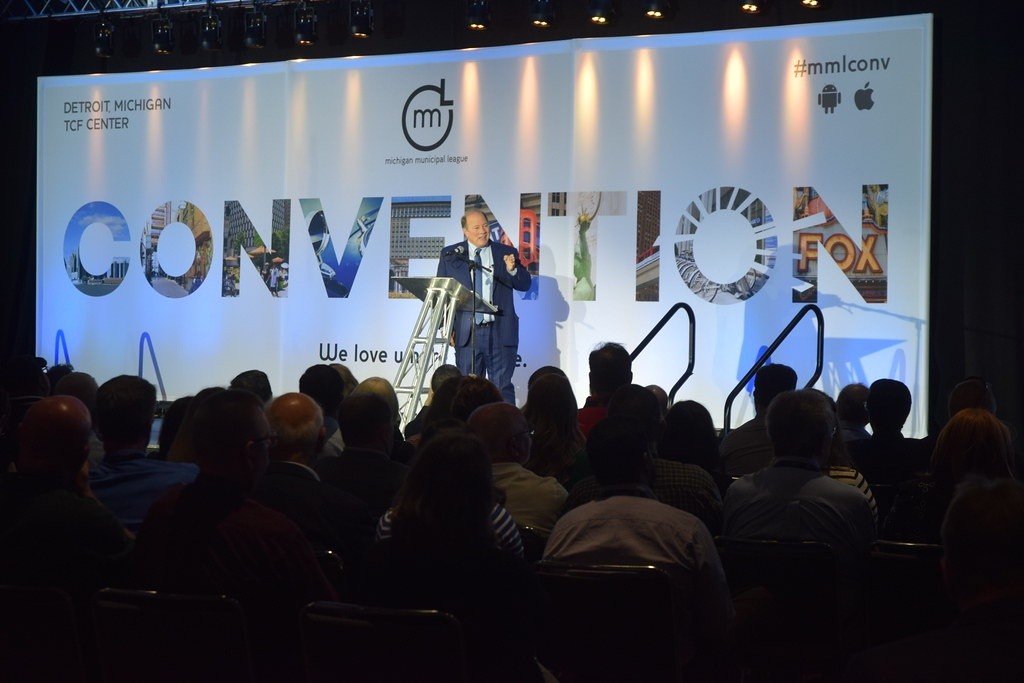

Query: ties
[474,248,484,325]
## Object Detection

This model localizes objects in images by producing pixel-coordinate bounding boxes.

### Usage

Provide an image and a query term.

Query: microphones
[443,245,466,256]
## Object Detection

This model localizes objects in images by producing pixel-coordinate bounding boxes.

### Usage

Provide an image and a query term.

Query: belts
[474,322,493,328]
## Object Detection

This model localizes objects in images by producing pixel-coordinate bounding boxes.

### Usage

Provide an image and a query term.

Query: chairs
[1,538,942,683]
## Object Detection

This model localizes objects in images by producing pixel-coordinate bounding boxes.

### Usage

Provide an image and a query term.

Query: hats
[300,365,348,409]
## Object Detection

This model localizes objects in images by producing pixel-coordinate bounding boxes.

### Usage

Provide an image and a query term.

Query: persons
[0,344,1024,683]
[431,211,532,407]
[222,266,240,298]
[259,262,289,297]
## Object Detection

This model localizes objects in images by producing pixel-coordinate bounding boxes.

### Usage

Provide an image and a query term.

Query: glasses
[381,412,400,425]
[506,424,536,447]
[241,429,279,453]
[817,420,837,440]
